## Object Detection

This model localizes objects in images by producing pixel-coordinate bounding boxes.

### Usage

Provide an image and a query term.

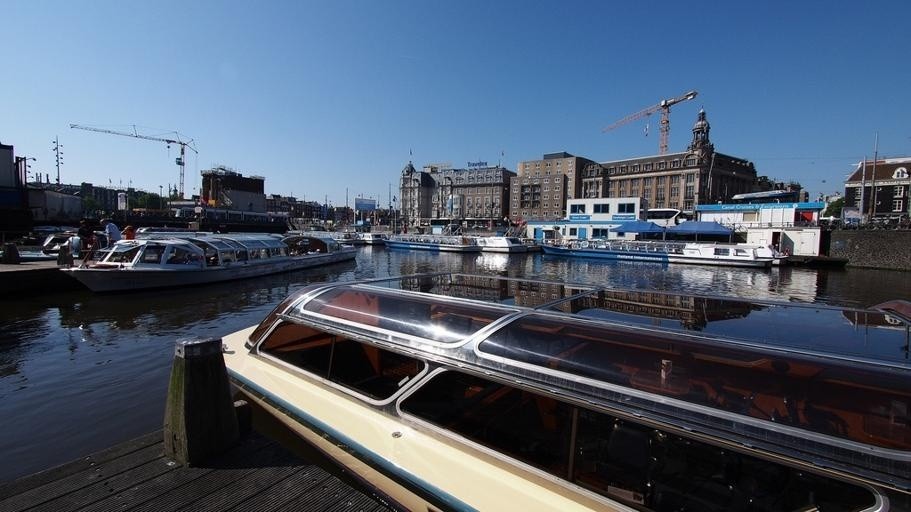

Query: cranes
[68,122,199,199]
[603,87,700,156]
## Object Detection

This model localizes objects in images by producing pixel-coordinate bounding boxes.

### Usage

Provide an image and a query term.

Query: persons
[77,217,94,260]
[100,219,121,250]
[183,247,320,265]
[120,226,136,240]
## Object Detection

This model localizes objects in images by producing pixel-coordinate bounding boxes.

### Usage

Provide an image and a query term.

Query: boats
[209,270,910,512]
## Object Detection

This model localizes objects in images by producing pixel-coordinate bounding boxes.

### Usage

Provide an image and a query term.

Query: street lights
[51,134,64,184]
[12,155,37,183]
[392,195,397,235]
